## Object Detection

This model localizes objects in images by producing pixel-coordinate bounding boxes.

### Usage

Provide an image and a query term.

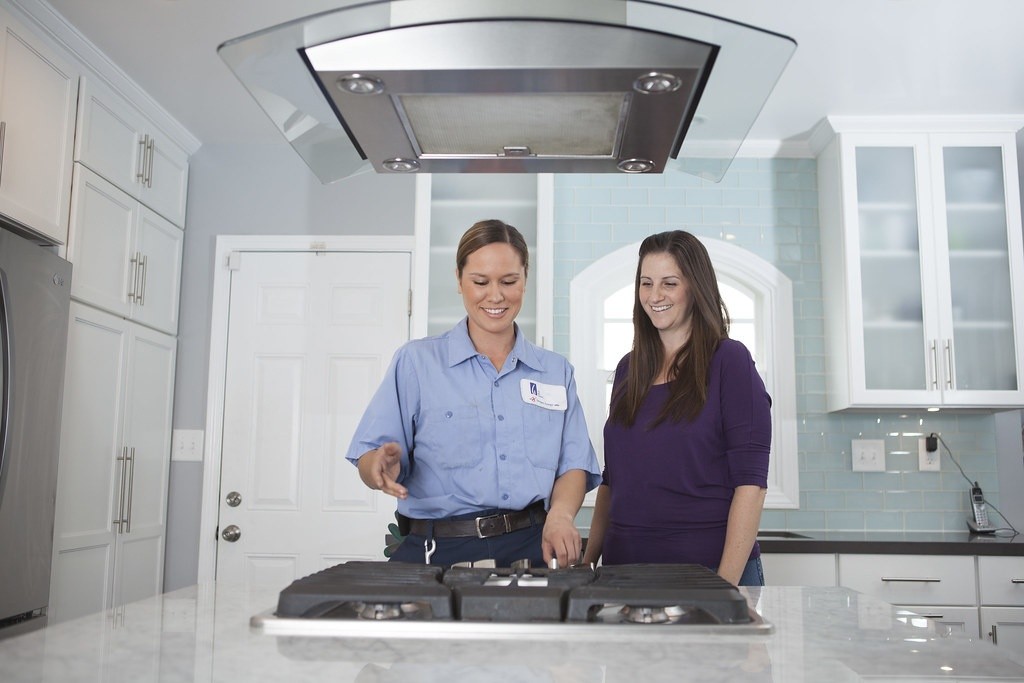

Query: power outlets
[919,438,940,472]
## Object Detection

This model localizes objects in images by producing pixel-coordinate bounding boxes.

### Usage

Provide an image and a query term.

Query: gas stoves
[250,559,775,640]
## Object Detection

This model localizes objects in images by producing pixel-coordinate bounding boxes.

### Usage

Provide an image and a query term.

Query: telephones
[965,481,998,535]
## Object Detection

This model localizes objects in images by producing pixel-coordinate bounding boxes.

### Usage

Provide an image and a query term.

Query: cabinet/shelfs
[807,111,1024,412]
[1,0,203,633]
[756,551,1024,664]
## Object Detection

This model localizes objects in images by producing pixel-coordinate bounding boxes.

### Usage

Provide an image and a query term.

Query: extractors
[214,0,799,183]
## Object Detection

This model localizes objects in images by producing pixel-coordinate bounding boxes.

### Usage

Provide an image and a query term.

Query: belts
[408,503,547,538]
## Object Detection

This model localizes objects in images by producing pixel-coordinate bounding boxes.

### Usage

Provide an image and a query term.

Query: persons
[581,231,769,586]
[345,220,604,576]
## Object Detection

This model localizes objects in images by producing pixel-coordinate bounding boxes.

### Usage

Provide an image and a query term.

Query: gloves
[384,509,407,556]
